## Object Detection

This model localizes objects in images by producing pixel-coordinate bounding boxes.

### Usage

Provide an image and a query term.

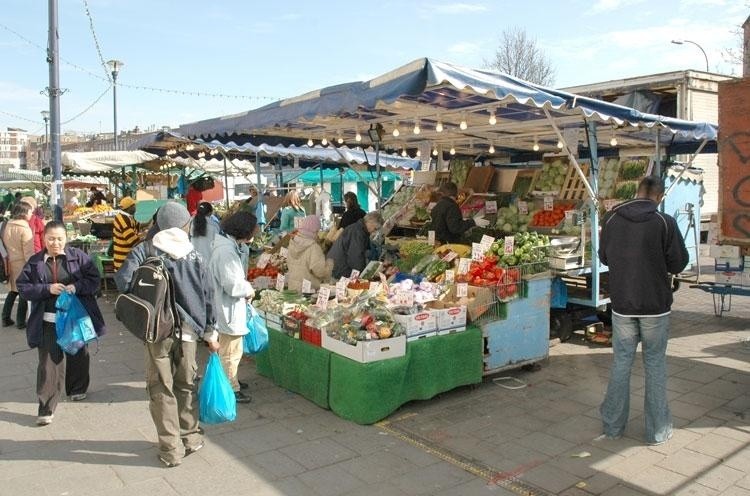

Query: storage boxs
[320,325,407,364]
[393,309,437,337]
[430,305,467,331]
[301,322,322,347]
[442,283,494,322]
[266,303,297,332]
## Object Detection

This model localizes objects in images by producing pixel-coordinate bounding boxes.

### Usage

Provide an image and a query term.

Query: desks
[96,255,114,300]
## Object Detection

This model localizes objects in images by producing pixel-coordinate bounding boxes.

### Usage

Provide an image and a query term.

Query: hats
[299,215,322,232]
[156,202,194,234]
[118,196,137,210]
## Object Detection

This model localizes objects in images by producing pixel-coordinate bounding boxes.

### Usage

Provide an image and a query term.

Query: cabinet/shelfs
[378,158,655,306]
[255,278,552,425]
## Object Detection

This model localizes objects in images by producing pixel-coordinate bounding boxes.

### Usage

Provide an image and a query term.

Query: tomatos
[533,204,573,226]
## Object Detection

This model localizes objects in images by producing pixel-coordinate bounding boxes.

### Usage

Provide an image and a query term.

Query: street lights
[672,38,710,72]
[40,111,51,150]
[106,60,123,150]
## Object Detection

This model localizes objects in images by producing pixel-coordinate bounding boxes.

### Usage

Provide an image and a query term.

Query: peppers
[485,231,549,274]
[456,256,518,299]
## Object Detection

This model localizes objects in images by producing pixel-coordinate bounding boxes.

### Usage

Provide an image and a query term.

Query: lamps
[322,135,327,145]
[488,114,497,125]
[307,135,313,147]
[190,141,194,151]
[166,148,170,155]
[170,147,173,155]
[173,146,176,154]
[556,140,563,149]
[450,147,455,155]
[609,137,618,146]
[186,142,190,151]
[489,145,494,154]
[338,134,343,143]
[401,148,407,156]
[533,141,539,151]
[367,123,385,143]
[413,122,420,134]
[392,126,400,136]
[460,120,468,130]
[356,131,361,141]
[432,147,438,156]
[178,143,182,152]
[435,121,443,132]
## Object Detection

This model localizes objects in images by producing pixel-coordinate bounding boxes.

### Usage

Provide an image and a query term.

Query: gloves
[474,214,490,228]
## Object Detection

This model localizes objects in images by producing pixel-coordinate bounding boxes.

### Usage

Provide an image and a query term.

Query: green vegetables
[615,181,637,200]
[622,160,647,179]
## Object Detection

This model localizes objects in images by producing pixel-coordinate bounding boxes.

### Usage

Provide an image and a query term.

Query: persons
[20,196,46,254]
[0,202,9,284]
[430,182,489,246]
[309,183,320,215]
[0,202,35,329]
[113,197,152,269]
[288,215,333,296]
[15,220,108,425]
[6,192,21,213]
[598,176,689,445]
[181,184,202,217]
[208,212,257,403]
[114,203,220,467]
[337,192,366,230]
[190,202,221,263]
[327,211,384,285]
[69,191,81,207]
[85,186,106,208]
[280,191,306,237]
[245,186,257,205]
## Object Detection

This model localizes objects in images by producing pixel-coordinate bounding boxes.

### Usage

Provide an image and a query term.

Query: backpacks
[114,239,184,367]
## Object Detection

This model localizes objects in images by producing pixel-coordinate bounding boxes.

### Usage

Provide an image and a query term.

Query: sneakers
[238,379,249,390]
[69,394,88,400]
[235,392,252,403]
[157,456,182,468]
[35,414,55,426]
[186,441,205,456]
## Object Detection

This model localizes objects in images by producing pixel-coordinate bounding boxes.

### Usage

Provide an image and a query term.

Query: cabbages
[496,203,535,233]
[535,160,568,191]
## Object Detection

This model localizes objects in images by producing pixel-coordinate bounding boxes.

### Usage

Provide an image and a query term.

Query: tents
[61,150,255,205]
[43,161,222,200]
[0,179,111,204]
[9,166,109,182]
[181,60,720,308]
[127,127,423,235]
